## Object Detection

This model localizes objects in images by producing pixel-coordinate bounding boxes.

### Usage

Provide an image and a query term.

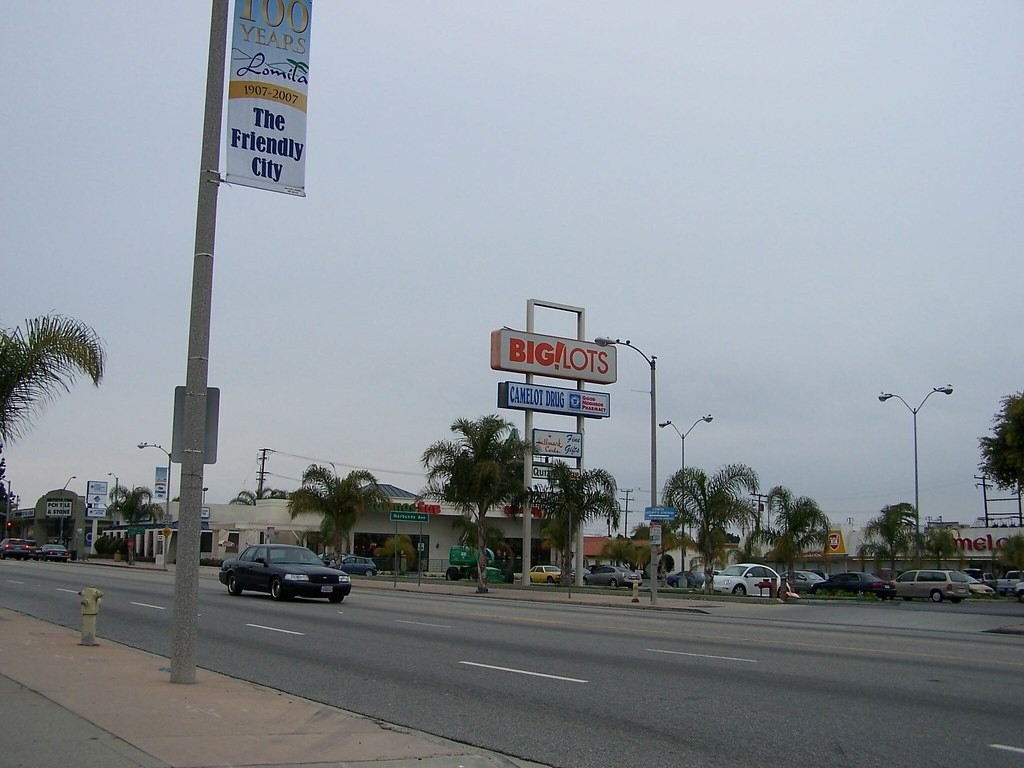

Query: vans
[890,569,972,603]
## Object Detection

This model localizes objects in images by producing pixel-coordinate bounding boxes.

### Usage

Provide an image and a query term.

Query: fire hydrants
[79,584,103,645]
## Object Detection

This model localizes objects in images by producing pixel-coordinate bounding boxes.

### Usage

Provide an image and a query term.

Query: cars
[1006,570,1024,602]
[34,544,70,563]
[219,544,352,603]
[778,570,825,594]
[808,571,897,600]
[666,563,782,598]
[513,565,562,583]
[583,566,642,587]
[964,575,996,598]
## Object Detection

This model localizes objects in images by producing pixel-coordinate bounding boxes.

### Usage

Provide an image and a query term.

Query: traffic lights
[7,521,11,530]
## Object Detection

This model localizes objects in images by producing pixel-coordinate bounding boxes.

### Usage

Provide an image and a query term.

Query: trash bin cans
[70,550,77,560]
[114,553,121,562]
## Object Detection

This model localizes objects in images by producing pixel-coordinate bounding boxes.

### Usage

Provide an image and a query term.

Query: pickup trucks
[966,568,1021,594]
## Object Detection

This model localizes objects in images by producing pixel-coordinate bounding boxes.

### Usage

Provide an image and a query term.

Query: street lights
[595,337,658,604]
[659,416,714,571]
[878,385,953,568]
[59,444,171,570]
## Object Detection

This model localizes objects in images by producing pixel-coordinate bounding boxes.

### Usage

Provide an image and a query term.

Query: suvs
[317,552,377,576]
[0,538,39,561]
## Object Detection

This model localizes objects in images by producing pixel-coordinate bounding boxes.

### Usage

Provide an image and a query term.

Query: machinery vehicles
[446,545,514,583]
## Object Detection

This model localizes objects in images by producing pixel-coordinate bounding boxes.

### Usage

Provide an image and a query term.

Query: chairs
[285,550,301,562]
